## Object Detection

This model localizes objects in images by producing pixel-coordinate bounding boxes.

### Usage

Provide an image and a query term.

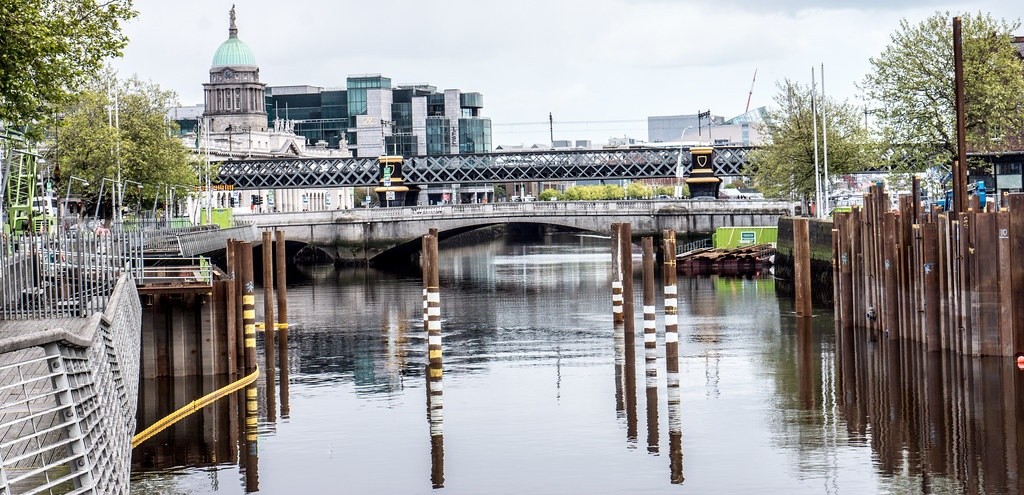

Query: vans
[27,196,64,216]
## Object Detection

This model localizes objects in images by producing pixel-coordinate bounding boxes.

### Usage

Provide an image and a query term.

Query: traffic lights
[77,201,82,213]
[231,197,234,206]
[69,203,74,213]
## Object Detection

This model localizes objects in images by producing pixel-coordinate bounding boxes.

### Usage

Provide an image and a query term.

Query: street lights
[94,177,123,219]
[152,181,213,225]
[62,175,90,225]
[676,125,694,198]
[121,179,144,202]
